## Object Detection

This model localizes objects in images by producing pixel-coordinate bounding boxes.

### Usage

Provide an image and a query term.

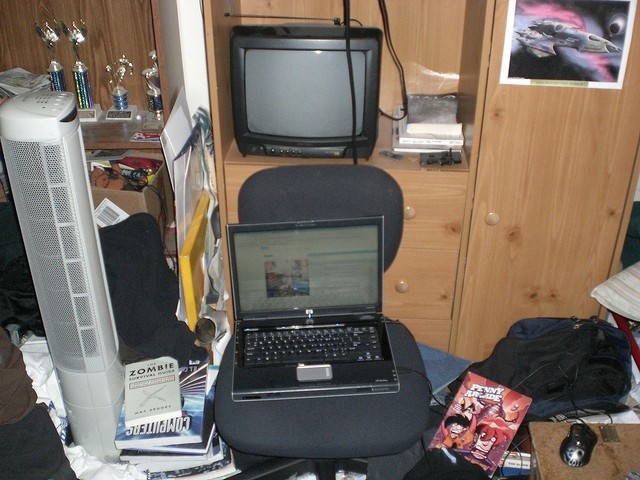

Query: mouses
[561,424,597,467]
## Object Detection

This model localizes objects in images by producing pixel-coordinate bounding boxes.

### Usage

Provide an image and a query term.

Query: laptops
[225,216,400,402]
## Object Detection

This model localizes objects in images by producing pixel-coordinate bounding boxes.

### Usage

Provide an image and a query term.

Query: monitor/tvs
[230,24,383,159]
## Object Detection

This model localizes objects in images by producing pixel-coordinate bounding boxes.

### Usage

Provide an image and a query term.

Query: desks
[529,419,640,480]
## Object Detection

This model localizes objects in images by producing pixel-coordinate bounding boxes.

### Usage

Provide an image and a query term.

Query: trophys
[106,54,138,121]
[141,50,164,121]
[34,19,67,92]
[60,19,102,122]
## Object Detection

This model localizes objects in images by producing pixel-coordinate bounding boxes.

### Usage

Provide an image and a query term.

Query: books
[114,355,242,480]
[391,91,464,155]
[433,370,533,478]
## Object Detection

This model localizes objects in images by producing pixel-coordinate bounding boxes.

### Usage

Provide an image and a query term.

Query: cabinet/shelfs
[202,2,493,356]
[0,0,183,257]
[448,1,639,365]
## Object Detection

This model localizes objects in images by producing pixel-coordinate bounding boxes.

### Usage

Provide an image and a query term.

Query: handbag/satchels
[448,315,633,426]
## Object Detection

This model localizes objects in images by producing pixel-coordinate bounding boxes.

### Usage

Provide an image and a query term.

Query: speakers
[1,91,126,463]
